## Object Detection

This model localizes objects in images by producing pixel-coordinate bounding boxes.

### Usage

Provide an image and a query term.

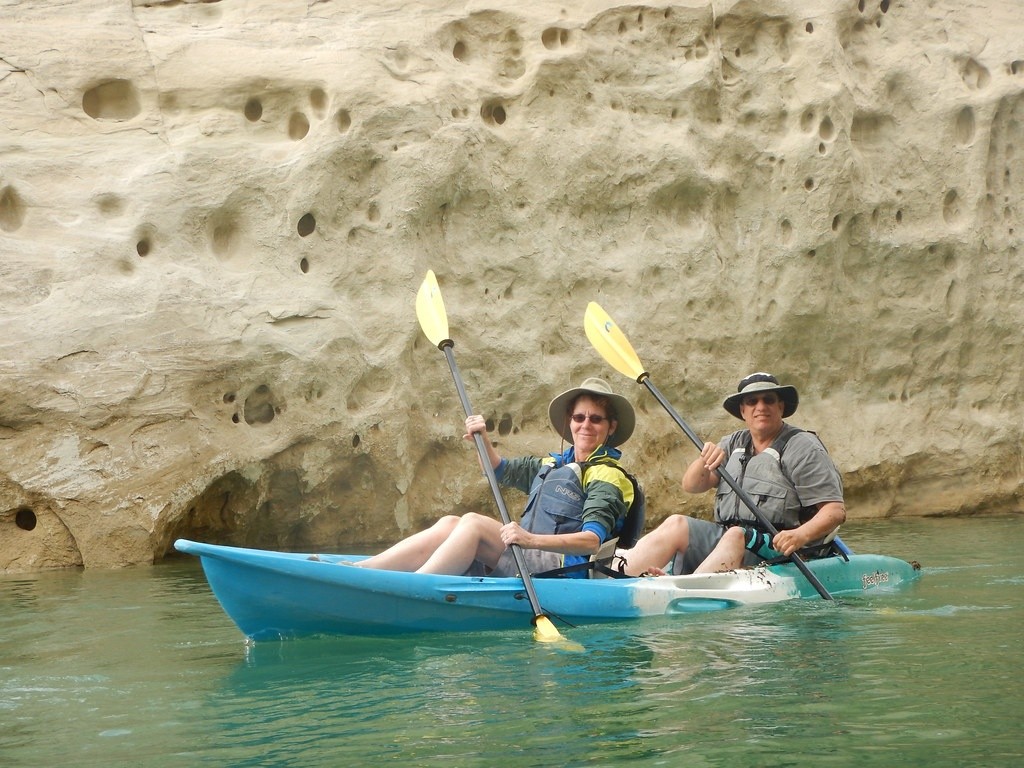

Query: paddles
[414,267,563,641]
[583,300,836,602]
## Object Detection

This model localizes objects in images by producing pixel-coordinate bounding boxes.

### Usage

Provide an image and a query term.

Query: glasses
[740,396,781,406]
[569,414,613,423]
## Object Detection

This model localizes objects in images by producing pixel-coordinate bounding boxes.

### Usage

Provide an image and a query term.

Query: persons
[607,373,847,579]
[306,378,634,579]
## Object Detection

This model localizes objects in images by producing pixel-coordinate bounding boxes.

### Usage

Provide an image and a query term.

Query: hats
[722,372,800,421]
[548,377,635,448]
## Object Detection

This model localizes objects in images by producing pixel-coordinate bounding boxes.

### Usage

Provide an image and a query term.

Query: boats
[171,531,922,654]
